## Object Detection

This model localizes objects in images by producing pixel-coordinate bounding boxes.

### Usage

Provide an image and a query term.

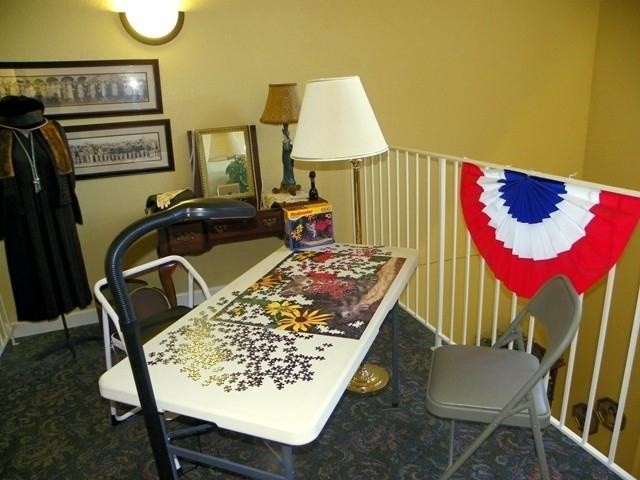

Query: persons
[0,75,147,104]
[68,135,161,164]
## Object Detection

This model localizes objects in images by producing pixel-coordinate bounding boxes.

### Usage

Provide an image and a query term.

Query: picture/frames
[572,398,626,435]
[1,58,176,181]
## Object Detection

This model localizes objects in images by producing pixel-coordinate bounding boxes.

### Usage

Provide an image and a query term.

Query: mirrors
[187,125,264,210]
